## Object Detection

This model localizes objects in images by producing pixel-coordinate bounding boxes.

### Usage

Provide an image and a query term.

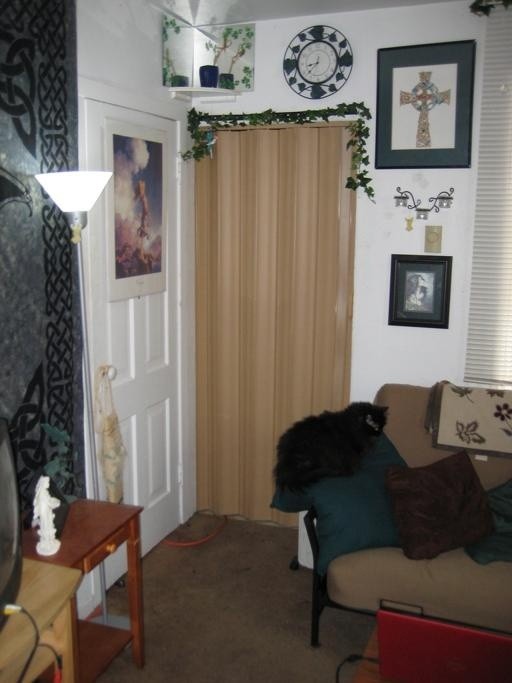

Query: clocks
[282,24,354,100]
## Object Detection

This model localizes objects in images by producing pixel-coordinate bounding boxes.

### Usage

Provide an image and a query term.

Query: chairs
[299,378,512,650]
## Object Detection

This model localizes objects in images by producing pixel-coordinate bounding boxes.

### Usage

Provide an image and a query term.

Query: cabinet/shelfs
[18,495,145,682]
[1,557,85,682]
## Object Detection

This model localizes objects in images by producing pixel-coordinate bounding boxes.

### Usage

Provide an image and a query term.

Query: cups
[170,75,189,87]
[199,65,218,88]
[219,73,234,89]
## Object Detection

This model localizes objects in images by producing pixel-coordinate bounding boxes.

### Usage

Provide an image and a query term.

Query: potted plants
[161,20,191,87]
[198,22,244,89]
[219,26,255,90]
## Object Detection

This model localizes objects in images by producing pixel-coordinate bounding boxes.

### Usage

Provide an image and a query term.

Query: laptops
[377,607,512,683]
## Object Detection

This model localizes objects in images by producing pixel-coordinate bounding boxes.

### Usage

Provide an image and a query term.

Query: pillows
[383,451,496,560]
[464,475,512,565]
[269,423,412,575]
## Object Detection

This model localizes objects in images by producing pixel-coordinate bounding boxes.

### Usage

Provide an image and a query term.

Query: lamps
[393,185,456,220]
[32,168,136,655]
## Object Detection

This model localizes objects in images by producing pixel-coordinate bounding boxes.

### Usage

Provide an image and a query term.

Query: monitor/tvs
[1,417,23,631]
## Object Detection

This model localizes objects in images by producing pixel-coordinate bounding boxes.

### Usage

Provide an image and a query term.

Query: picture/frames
[100,116,169,301]
[374,38,476,169]
[387,253,453,329]
